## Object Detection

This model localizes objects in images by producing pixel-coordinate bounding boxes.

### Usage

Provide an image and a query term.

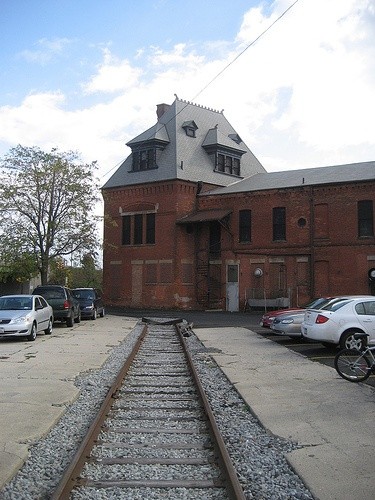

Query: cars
[269,296,336,341]
[0,294,55,341]
[300,295,375,353]
[260,296,336,328]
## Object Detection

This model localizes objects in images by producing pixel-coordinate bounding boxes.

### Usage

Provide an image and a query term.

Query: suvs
[69,286,106,320]
[31,284,82,327]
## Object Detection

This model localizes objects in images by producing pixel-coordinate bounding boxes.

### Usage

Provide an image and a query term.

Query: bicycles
[334,332,375,383]
